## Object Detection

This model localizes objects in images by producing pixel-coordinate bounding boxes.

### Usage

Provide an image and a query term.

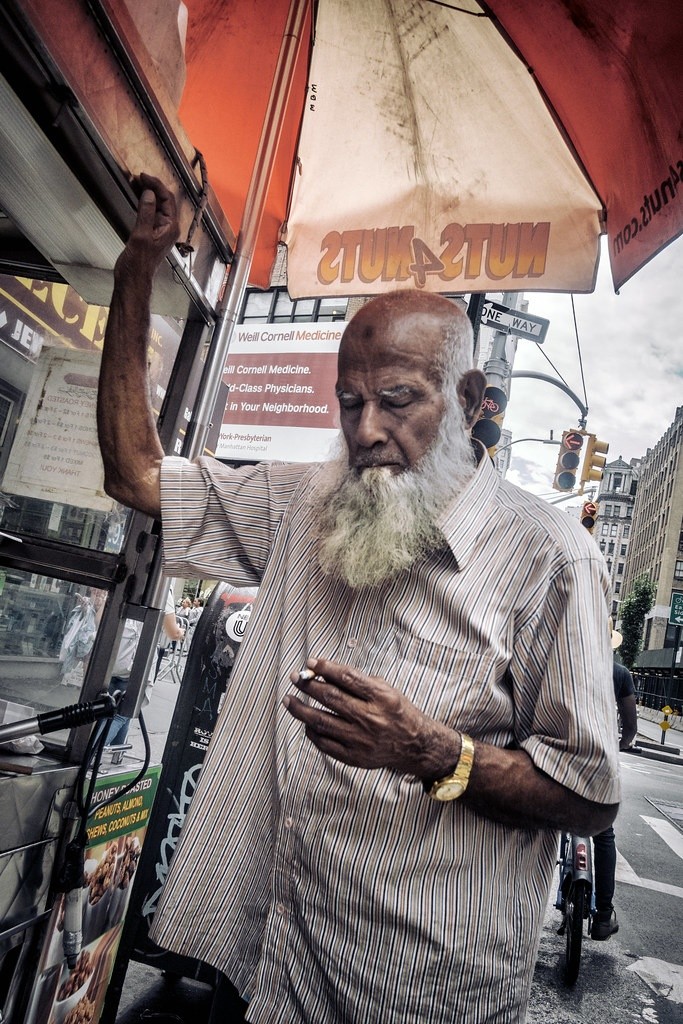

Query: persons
[171,597,203,657]
[592,616,637,941]
[96,172,624,1023]
[81,581,185,776]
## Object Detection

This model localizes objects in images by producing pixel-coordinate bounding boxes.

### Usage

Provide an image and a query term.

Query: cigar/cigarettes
[299,669,317,681]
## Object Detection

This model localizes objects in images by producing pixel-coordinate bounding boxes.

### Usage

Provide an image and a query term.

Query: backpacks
[108,624,141,680]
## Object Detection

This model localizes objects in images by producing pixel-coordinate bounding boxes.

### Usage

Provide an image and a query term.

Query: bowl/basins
[52,960,96,1024]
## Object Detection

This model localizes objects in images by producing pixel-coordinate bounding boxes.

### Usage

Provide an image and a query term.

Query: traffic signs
[478,301,550,342]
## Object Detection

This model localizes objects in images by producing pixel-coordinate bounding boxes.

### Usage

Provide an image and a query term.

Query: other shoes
[591,915,618,940]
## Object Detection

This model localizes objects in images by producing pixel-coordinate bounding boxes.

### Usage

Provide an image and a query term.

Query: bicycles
[557,834,620,988]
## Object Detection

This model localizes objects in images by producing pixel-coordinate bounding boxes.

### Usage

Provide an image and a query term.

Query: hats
[609,614,623,649]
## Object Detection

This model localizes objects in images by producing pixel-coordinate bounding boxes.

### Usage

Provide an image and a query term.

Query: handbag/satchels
[59,602,97,675]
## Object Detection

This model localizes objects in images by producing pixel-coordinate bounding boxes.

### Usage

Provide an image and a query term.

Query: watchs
[425,729,474,801]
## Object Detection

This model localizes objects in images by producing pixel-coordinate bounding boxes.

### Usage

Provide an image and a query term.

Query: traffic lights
[471,387,508,450]
[580,437,610,483]
[579,500,600,536]
[553,430,584,493]
